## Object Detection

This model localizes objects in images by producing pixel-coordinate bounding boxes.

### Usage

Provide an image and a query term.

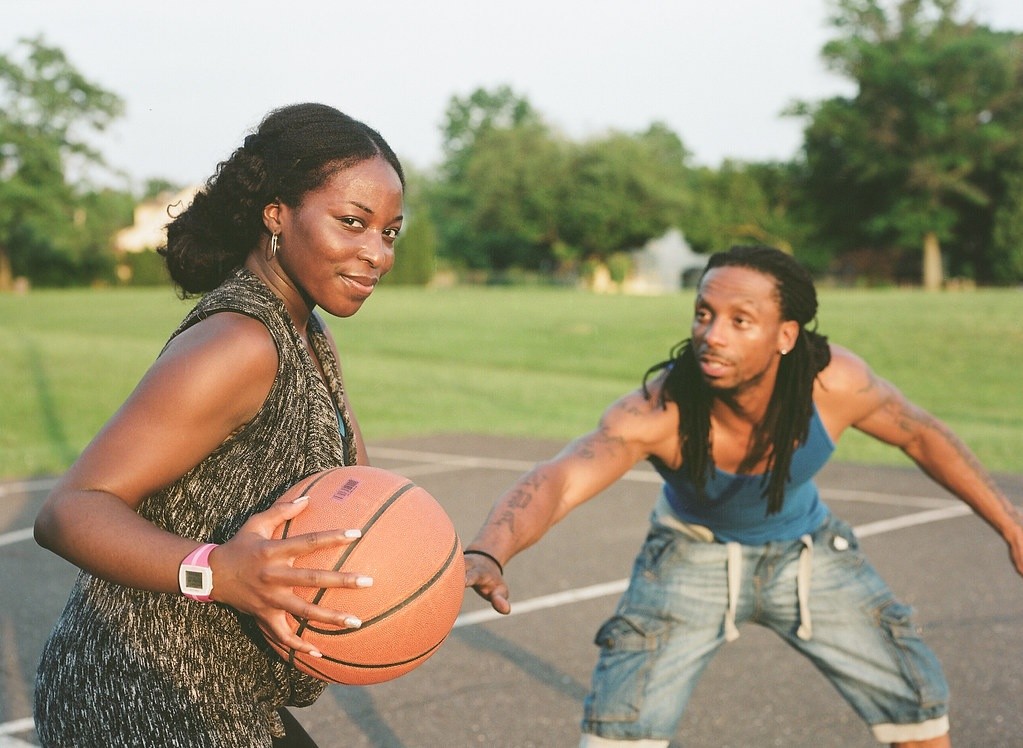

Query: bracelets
[463,549,503,575]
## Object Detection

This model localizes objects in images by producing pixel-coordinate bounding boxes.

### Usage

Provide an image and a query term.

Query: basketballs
[256,463,469,688]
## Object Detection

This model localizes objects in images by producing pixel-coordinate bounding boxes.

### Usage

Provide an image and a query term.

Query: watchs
[178,543,220,602]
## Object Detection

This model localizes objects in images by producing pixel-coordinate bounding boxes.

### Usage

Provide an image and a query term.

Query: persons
[463,245,1023,748]
[31,103,406,748]
